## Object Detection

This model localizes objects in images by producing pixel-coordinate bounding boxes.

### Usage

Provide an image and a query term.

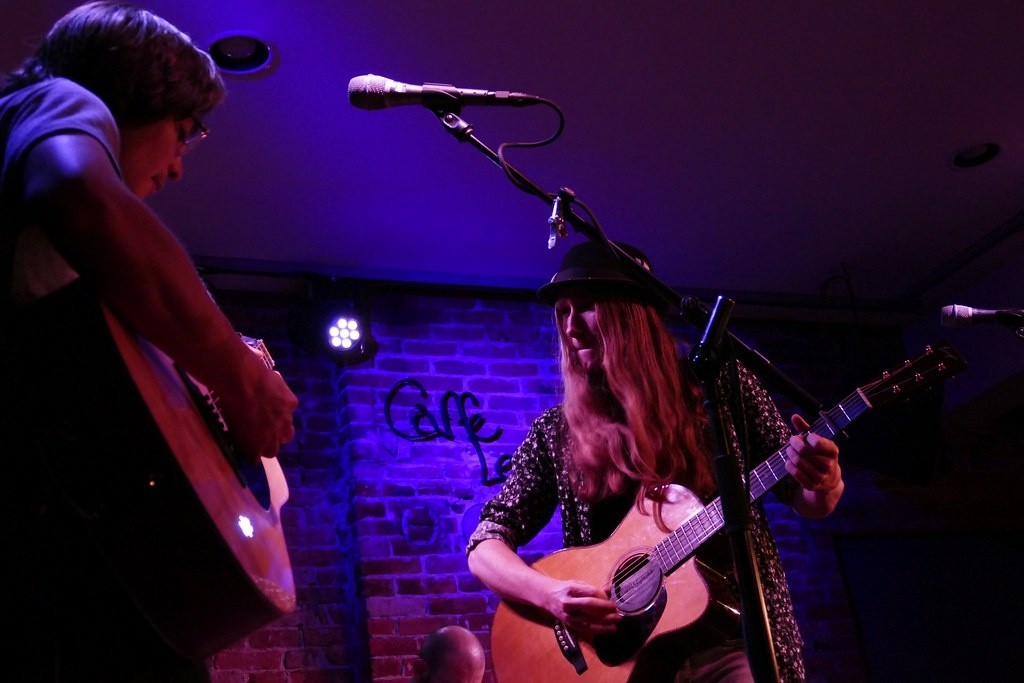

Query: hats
[534,242,670,306]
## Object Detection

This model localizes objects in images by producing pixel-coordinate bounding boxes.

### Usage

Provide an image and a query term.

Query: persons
[410,625,485,683]
[466,238,844,683]
[0,0,299,468]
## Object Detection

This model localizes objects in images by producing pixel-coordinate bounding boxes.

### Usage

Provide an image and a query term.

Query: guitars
[490,341,973,683]
[25,276,299,670]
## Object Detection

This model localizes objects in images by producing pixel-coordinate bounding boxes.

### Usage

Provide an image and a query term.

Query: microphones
[940,303,1024,330]
[348,73,539,111]
